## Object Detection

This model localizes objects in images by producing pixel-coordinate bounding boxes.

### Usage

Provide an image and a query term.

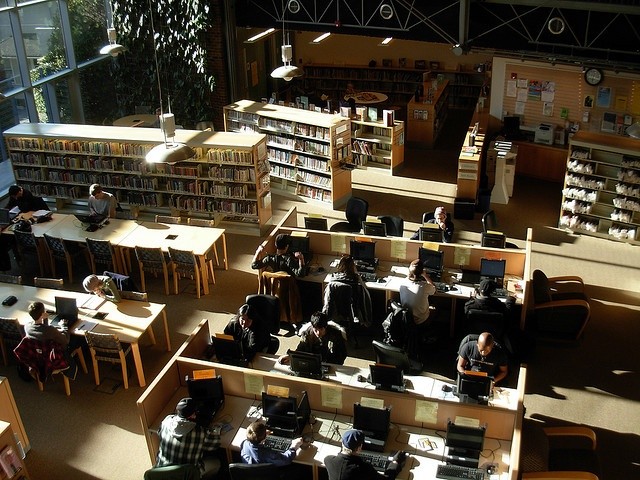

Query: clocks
[584,69,601,84]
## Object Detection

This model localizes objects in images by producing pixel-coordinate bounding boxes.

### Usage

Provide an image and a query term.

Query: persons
[457,332,507,384]
[83,273,121,304]
[88,183,116,219]
[252,233,305,292]
[400,259,436,326]
[294,311,347,364]
[223,303,259,360]
[3,185,34,213]
[321,255,372,324]
[24,301,70,347]
[154,398,221,471]
[325,428,404,480]
[240,421,303,466]
[409,206,455,244]
[464,278,517,323]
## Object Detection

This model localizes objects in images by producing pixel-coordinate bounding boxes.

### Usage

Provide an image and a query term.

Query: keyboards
[355,271,377,282]
[435,465,484,480]
[491,288,508,298]
[420,281,446,293]
[351,451,391,473]
[264,435,292,452]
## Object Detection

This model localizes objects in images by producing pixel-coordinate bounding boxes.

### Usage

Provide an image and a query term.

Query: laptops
[74,214,106,223]
[51,297,77,328]
[1,207,17,224]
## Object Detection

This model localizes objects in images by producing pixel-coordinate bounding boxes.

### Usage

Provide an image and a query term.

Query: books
[351,140,373,156]
[7,135,254,165]
[226,108,331,141]
[16,182,257,216]
[227,121,329,157]
[10,151,256,183]
[265,147,329,172]
[14,166,249,200]
[372,124,391,164]
[269,164,331,189]
[257,91,395,127]
[350,152,371,168]
[297,185,332,203]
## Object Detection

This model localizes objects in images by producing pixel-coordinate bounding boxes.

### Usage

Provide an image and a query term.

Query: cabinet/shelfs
[559,130,636,247]
[238,29,280,95]
[261,95,405,174]
[458,78,489,201]
[3,122,273,237]
[222,99,353,210]
[304,65,486,112]
[406,76,451,143]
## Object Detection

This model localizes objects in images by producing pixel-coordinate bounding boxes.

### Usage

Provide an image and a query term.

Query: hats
[434,207,447,219]
[343,430,364,450]
[176,398,195,418]
[83,274,100,292]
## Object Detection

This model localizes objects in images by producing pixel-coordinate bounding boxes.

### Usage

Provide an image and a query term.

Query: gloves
[393,451,406,463]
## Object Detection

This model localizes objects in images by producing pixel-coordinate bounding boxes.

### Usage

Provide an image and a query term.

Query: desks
[323,254,393,289]
[267,352,354,386]
[148,381,256,467]
[431,380,517,412]
[5,212,68,239]
[44,215,142,274]
[453,269,524,342]
[315,413,424,480]
[264,243,332,281]
[231,396,337,480]
[349,367,435,400]
[385,265,457,302]
[0,268,172,388]
[121,222,229,294]
[208,342,279,374]
[409,426,511,480]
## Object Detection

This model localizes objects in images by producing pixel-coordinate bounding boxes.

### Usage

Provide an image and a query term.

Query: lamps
[141,1,196,163]
[270,0,299,82]
[100,0,123,56]
[626,120,640,139]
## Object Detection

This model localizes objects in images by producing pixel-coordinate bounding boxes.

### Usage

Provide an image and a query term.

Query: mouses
[358,375,363,382]
[317,267,325,272]
[279,359,284,364]
[442,385,448,392]
[487,464,495,474]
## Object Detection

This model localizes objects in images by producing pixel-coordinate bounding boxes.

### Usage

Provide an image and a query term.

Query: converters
[46,310,54,314]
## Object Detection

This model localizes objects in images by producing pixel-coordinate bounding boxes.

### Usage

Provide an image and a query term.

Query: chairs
[325,279,364,331]
[156,215,179,224]
[85,332,133,391]
[481,210,517,248]
[380,214,404,235]
[42,236,74,283]
[133,246,168,297]
[304,325,345,365]
[1,272,24,285]
[327,198,367,233]
[381,302,415,340]
[103,272,129,300]
[466,309,500,333]
[520,424,599,478]
[9,237,46,278]
[455,333,503,358]
[169,247,200,298]
[87,241,120,281]
[188,218,220,285]
[241,294,280,352]
[24,336,90,396]
[119,290,147,301]
[85,241,114,275]
[533,270,590,339]
[32,276,67,289]
[416,211,451,230]
[229,464,272,480]
[257,273,294,325]
[367,341,410,374]
[144,464,199,480]
[0,319,22,358]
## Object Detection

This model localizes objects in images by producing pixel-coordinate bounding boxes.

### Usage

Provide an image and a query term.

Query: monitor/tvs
[289,236,309,255]
[262,392,296,425]
[288,350,324,381]
[369,364,404,393]
[419,227,444,242]
[480,258,506,283]
[481,232,505,248]
[446,421,485,459]
[212,336,244,368]
[353,404,390,438]
[377,277,383,283]
[304,217,327,231]
[363,222,386,237]
[185,376,224,407]
[418,246,443,269]
[350,240,375,260]
[457,373,491,404]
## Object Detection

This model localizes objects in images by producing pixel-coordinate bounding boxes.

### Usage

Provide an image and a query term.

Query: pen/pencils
[250,410,256,416]
[78,324,85,330]
